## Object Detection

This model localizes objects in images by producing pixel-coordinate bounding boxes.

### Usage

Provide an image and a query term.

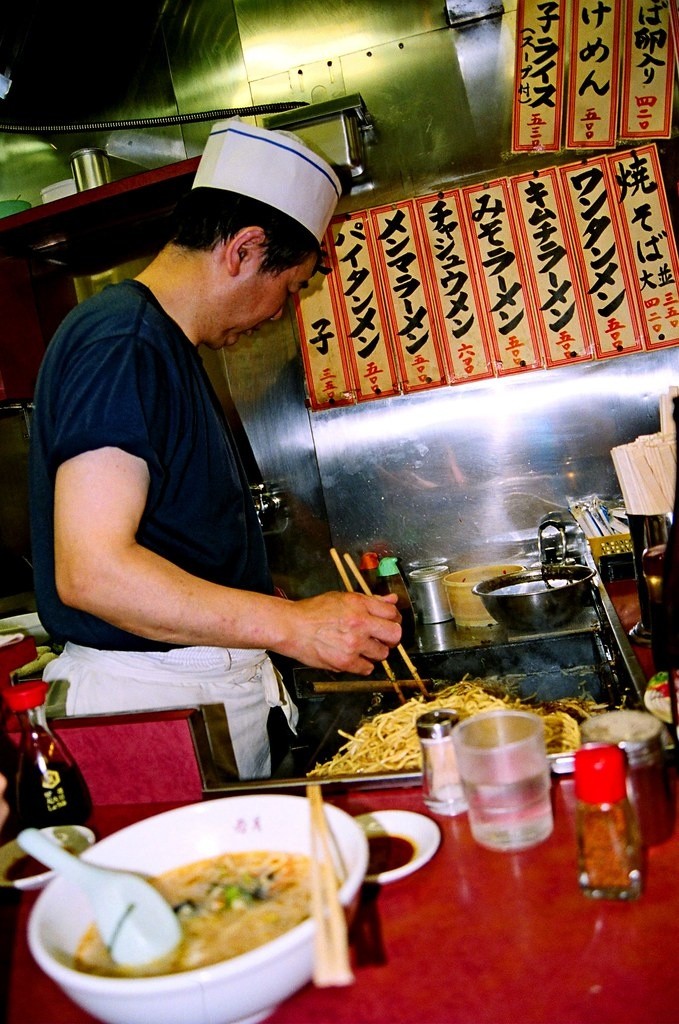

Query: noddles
[75,850,325,977]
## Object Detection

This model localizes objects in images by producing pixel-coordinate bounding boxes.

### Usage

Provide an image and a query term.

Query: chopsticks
[609,432,677,515]
[305,785,353,987]
[331,548,432,704]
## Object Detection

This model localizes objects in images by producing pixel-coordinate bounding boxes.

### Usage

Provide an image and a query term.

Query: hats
[191,116,342,245]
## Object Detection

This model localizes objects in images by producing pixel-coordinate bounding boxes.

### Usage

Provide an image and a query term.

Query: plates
[0,825,96,892]
[354,809,441,884]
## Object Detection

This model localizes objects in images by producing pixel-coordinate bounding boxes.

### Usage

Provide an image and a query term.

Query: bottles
[416,709,469,816]
[357,552,380,596]
[572,742,642,901]
[409,566,452,625]
[2,681,93,825]
[376,557,415,618]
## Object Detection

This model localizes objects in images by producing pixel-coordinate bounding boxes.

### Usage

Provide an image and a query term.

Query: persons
[31,118,402,780]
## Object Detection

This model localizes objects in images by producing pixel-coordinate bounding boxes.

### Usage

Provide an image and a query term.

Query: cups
[451,710,553,852]
[625,511,674,631]
[579,711,671,845]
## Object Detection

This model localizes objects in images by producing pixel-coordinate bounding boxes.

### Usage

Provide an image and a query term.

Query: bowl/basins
[40,178,77,204]
[0,200,31,219]
[27,795,369,1024]
[471,564,596,632]
[443,564,523,628]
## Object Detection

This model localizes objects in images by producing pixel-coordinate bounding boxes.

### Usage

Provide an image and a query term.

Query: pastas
[305,681,579,777]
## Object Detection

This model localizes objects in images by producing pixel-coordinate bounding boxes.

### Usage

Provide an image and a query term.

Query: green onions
[206,870,260,911]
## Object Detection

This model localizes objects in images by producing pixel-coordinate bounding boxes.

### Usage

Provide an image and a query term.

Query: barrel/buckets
[68,147,111,192]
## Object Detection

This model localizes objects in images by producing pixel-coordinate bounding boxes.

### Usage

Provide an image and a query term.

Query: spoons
[15,828,183,967]
